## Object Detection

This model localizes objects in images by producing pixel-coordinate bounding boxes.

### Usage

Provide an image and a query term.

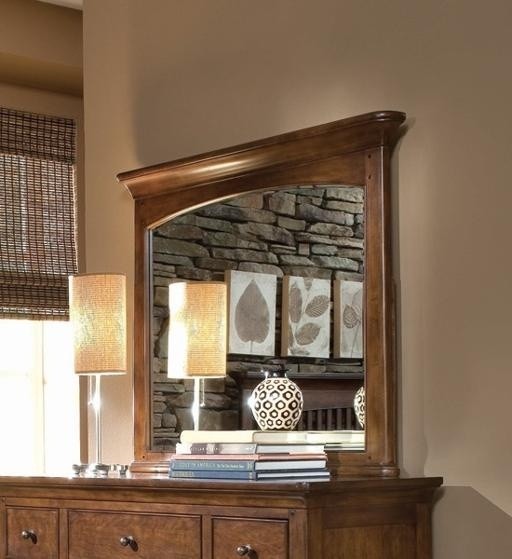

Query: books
[167,429,330,484]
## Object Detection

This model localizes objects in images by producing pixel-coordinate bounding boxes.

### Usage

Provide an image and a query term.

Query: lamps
[68,273,127,463]
[166,282,227,431]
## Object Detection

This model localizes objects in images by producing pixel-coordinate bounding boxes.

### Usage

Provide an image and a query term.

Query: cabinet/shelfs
[0,475,443,559]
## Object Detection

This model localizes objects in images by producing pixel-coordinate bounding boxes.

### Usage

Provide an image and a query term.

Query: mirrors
[114,109,406,477]
